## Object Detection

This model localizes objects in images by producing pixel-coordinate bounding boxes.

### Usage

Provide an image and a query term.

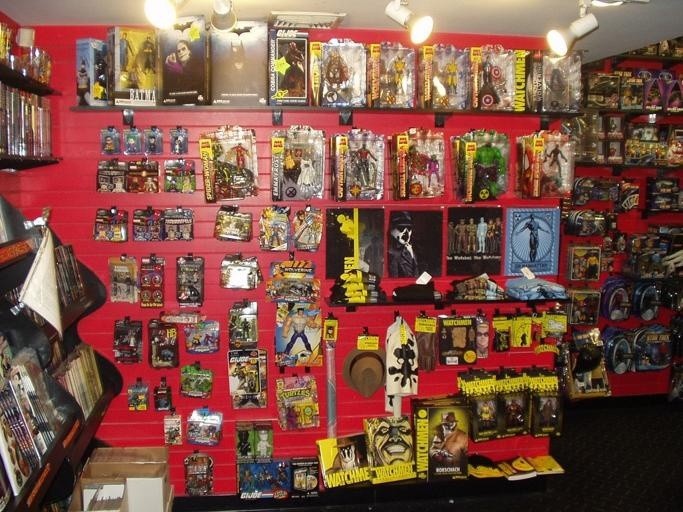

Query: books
[0,81,53,156]
[0,243,104,511]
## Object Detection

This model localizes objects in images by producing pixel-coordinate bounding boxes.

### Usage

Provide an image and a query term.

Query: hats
[391,211,414,230]
[440,413,458,424]
[333,438,354,448]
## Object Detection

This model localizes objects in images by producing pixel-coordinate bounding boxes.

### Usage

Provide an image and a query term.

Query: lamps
[210,0,237,33]
[547,0,599,56]
[144,0,190,29]
[385,0,434,45]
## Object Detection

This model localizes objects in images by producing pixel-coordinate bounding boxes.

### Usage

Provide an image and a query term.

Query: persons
[282,42,578,200]
